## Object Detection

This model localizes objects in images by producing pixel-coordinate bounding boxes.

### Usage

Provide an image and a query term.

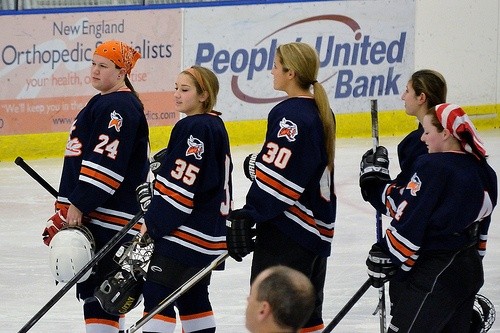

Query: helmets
[48,224,95,285]
[472,293,490,332]
[94,268,143,315]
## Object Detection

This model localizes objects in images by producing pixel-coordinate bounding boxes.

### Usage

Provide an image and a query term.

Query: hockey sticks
[322,278,374,333]
[18,209,145,333]
[370,99,388,333]
[15,156,58,198]
[118,235,257,333]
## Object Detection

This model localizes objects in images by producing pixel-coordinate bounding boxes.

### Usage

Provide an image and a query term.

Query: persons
[245,266,315,333]
[367,103,497,333]
[42,41,150,333]
[225,43,336,333]
[136,66,233,333]
[360,69,446,218]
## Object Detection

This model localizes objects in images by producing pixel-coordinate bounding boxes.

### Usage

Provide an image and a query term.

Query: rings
[74,220,76,222]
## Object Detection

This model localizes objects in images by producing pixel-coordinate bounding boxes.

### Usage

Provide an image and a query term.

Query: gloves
[41,207,67,245]
[242,153,258,178]
[366,244,397,288]
[359,147,392,203]
[226,220,256,261]
